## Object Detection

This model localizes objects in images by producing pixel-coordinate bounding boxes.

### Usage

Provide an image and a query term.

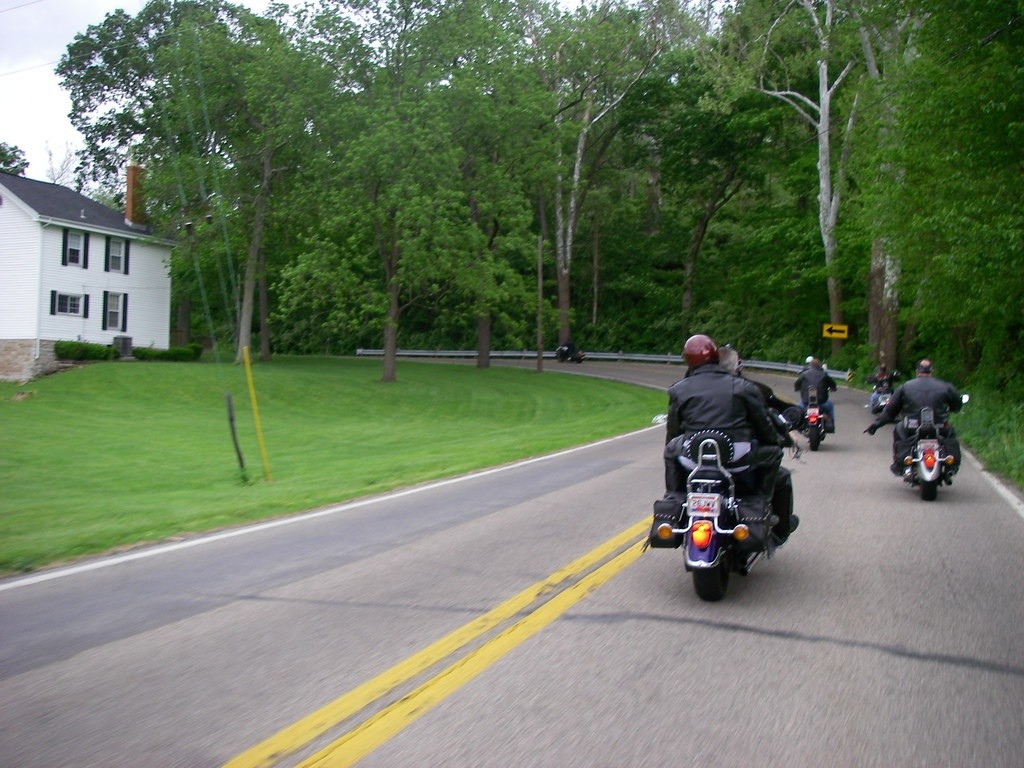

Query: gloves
[863,424,878,435]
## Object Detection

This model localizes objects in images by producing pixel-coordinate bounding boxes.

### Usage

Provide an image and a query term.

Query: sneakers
[775,515,799,544]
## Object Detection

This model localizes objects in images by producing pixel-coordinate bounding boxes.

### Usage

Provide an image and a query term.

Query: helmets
[806,356,814,363]
[682,335,720,366]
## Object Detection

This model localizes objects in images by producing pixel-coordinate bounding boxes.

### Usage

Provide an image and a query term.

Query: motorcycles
[865,371,899,415]
[556,345,586,363]
[794,387,830,451]
[650,348,804,601]
[879,391,970,501]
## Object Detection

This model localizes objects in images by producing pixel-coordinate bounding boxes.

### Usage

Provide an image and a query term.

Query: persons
[863,358,963,476]
[563,338,578,360]
[664,335,836,546]
